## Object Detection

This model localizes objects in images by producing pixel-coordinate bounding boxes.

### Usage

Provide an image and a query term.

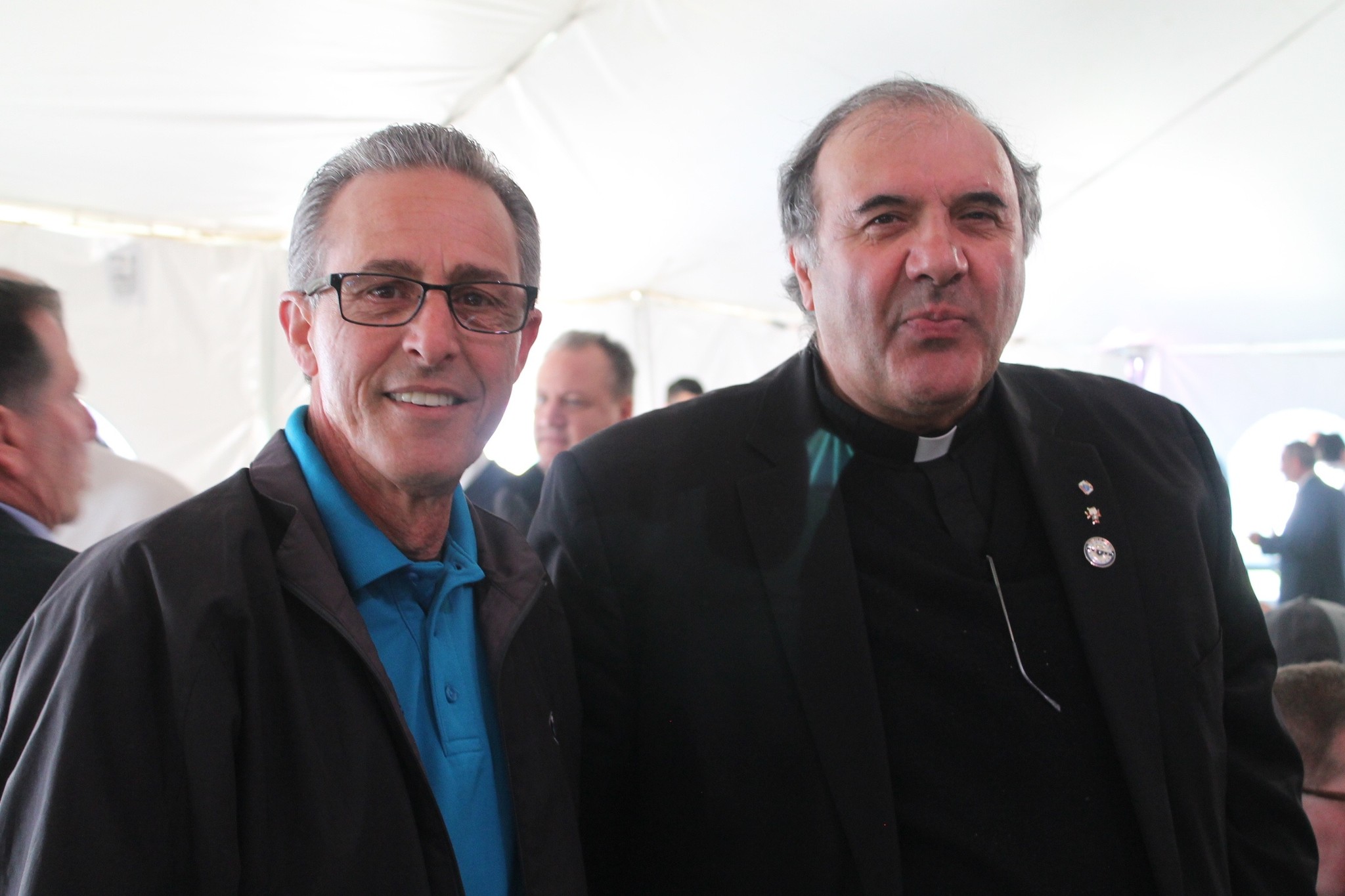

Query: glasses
[307,272,538,334]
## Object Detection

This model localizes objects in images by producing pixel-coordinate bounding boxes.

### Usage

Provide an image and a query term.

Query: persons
[0,124,546,896]
[526,77,1318,896]
[1,269,702,663]
[1243,433,1345,896]
